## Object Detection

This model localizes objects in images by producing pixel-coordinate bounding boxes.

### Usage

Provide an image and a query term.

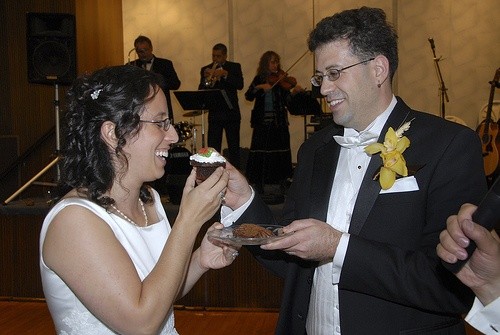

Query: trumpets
[203,60,220,88]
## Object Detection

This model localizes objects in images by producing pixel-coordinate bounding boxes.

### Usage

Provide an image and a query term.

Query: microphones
[442,177,500,273]
[428,37,435,48]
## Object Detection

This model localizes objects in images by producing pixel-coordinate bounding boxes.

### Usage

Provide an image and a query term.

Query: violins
[266,69,309,98]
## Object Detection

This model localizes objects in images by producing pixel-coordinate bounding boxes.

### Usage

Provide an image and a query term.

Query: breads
[232,223,274,238]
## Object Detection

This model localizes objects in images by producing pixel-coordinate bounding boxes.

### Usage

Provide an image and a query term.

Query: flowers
[363,117,417,189]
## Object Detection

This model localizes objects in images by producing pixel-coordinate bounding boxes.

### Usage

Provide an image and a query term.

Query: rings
[219,192,224,196]
[233,251,239,256]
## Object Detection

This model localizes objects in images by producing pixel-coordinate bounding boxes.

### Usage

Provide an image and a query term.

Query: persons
[124,35,181,126]
[245,50,294,193]
[196,6,487,335]
[40,65,242,335]
[437,203,500,335]
[198,43,244,172]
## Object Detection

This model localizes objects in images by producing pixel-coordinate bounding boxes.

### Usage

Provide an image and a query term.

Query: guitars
[477,68,500,178]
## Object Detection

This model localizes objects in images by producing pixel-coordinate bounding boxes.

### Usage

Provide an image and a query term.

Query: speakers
[26,11,76,84]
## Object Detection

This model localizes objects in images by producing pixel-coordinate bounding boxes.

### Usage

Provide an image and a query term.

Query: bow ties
[333,131,379,150]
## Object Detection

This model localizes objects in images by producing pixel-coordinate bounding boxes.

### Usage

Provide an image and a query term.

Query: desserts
[189,147,227,183]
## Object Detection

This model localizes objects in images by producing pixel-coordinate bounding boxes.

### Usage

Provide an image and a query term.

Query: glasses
[139,118,172,131]
[310,58,375,87]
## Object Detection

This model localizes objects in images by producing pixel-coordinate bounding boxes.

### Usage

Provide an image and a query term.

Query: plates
[207,224,294,245]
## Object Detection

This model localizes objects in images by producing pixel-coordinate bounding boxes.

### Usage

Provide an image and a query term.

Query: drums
[173,121,194,141]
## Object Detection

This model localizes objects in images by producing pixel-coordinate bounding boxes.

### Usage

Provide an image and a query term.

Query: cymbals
[182,110,208,117]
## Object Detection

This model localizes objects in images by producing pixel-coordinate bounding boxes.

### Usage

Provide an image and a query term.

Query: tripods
[2,84,73,204]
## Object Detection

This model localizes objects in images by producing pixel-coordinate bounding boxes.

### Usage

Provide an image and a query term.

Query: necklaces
[111,198,148,226]
